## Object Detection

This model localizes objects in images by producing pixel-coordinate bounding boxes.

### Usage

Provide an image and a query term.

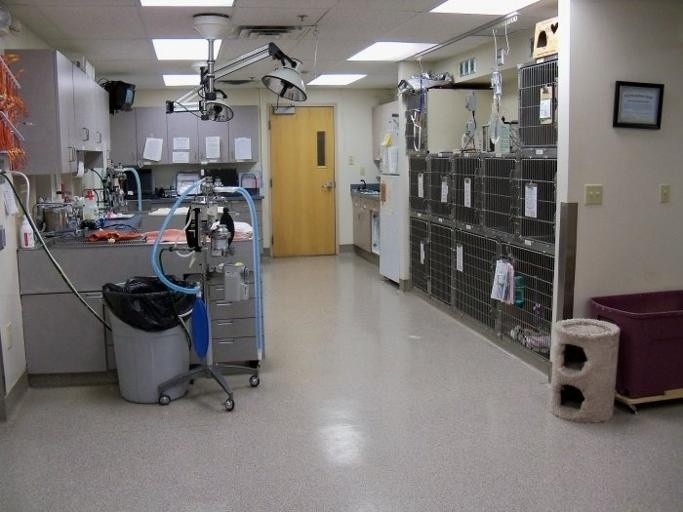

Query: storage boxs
[585,286,682,415]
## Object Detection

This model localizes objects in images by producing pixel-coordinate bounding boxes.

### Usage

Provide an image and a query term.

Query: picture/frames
[612,79,665,131]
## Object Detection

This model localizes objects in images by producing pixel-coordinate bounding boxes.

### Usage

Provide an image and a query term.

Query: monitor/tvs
[104,80,136,112]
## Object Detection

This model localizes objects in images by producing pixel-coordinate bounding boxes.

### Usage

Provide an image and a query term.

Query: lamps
[163,12,311,124]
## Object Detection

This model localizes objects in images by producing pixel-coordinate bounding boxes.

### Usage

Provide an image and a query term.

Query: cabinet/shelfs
[4,48,78,176]
[125,199,263,256]
[102,299,123,375]
[164,105,228,165]
[96,83,110,153]
[136,107,164,166]
[110,107,136,166]
[350,192,379,256]
[72,64,99,151]
[186,272,262,371]
[229,106,259,163]
[18,291,105,378]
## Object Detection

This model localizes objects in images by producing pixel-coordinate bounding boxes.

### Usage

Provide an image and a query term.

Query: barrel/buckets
[43,206,68,232]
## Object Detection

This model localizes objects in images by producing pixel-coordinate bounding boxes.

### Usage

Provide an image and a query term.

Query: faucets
[359,179,367,189]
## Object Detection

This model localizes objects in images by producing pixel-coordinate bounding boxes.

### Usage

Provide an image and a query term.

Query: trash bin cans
[103,276,196,404]
